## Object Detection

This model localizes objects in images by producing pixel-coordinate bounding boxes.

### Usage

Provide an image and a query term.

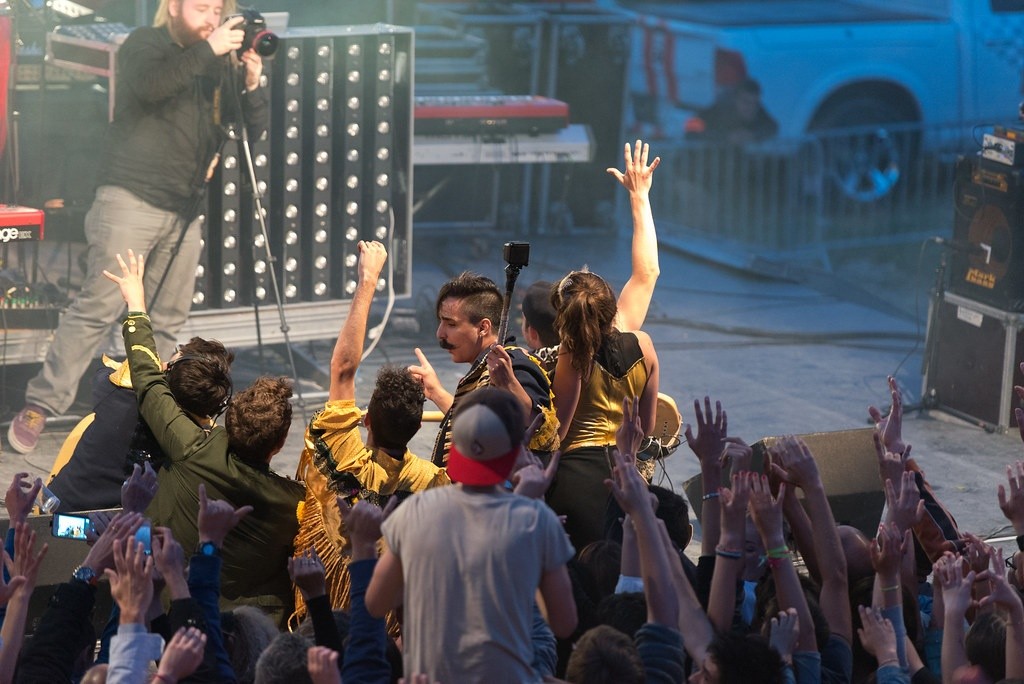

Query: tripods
[867,245,994,435]
[147,47,307,433]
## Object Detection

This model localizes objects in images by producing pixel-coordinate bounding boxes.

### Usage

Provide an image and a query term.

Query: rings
[111,526,118,532]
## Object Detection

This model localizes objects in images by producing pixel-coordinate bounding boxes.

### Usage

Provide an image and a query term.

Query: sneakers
[7,403,47,455]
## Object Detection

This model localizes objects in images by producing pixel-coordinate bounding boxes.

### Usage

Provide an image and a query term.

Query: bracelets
[878,584,900,593]
[702,492,719,502]
[715,547,744,558]
[1005,620,1024,626]
[879,658,900,664]
[765,545,790,563]
[151,673,169,684]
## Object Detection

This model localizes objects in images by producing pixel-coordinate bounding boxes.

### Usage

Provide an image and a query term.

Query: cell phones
[52,512,97,542]
[132,518,153,565]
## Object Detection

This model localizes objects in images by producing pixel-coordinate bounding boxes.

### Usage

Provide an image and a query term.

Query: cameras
[223,10,280,61]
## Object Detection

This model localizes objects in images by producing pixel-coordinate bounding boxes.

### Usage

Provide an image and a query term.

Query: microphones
[930,237,974,256]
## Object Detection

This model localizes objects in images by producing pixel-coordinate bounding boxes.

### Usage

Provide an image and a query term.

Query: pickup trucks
[590,0,1024,207]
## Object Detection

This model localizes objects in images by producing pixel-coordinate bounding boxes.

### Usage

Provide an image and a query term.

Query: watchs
[195,539,225,558]
[71,564,98,588]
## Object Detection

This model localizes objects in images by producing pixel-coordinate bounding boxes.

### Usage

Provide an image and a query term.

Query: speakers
[681,428,887,549]
[947,154,1024,314]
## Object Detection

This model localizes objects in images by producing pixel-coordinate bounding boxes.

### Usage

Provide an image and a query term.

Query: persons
[546,269,661,573]
[673,47,779,239]
[98,244,307,622]
[406,272,561,487]
[530,363,1024,684]
[522,136,662,386]
[363,387,576,683]
[291,237,451,655]
[5,0,273,455]
[0,335,406,684]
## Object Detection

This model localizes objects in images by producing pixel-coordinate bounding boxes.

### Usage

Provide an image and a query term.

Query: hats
[522,281,557,331]
[446,386,524,486]
[109,357,134,389]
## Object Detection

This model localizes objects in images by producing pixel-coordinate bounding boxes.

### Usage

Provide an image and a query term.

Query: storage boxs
[917,287,1024,437]
